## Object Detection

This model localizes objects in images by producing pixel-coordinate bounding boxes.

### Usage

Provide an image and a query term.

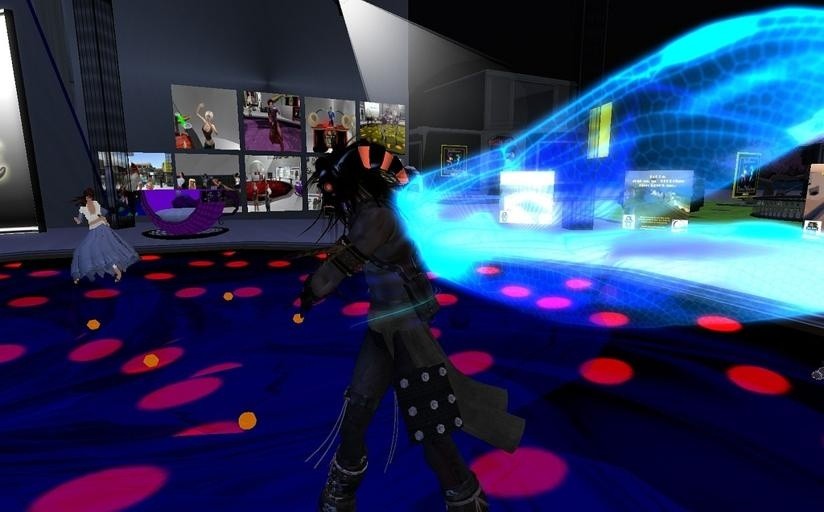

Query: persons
[328,106,334,126]
[259,99,285,152]
[124,168,164,190]
[70,188,140,284]
[252,183,272,212]
[197,104,218,148]
[290,139,490,511]
[177,171,241,190]
[380,111,400,126]
